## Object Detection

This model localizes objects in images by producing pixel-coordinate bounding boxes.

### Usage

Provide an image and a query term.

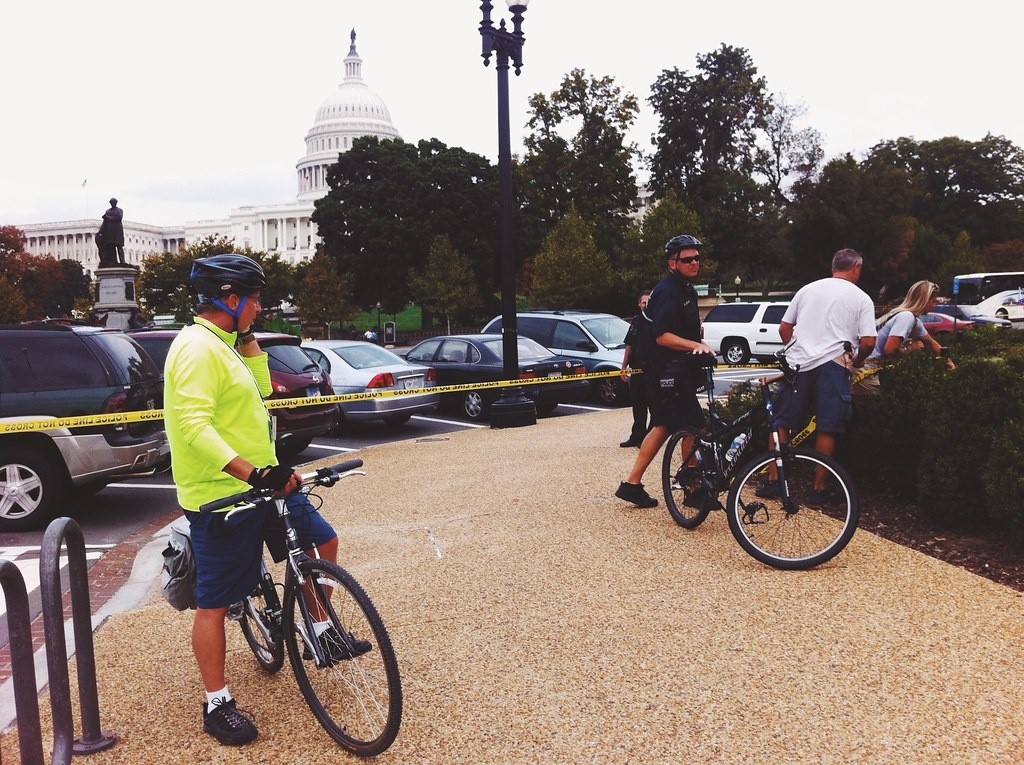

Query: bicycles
[198,458,403,757]
[662,338,861,571]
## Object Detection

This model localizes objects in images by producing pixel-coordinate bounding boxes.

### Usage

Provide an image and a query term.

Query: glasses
[670,255,700,263]
[246,295,263,304]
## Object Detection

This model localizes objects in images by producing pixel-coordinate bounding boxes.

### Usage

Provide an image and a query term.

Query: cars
[398,333,590,423]
[919,312,976,336]
[979,289,1024,320]
[301,340,440,437]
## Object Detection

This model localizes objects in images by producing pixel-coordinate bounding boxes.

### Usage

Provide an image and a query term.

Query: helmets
[190,255,267,304]
[665,235,702,257]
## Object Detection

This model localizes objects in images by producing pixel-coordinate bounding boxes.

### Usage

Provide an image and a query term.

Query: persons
[101,199,127,265]
[757,249,878,504]
[614,235,723,512]
[850,279,958,396]
[164,254,372,749]
[349,324,384,345]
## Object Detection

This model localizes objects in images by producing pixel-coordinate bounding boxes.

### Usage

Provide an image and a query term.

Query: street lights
[377,302,383,346]
[477,1,540,432]
[735,276,741,302]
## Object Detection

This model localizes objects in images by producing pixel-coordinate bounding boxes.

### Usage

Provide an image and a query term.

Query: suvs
[700,302,792,365]
[0,318,173,531]
[481,310,632,406]
[126,330,340,455]
[930,305,1012,330]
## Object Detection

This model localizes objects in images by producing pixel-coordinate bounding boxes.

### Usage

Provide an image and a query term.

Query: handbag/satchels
[162,524,198,613]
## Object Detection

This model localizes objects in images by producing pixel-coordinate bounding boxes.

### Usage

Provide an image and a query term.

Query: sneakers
[320,628,371,666]
[684,491,722,511]
[202,698,259,744]
[615,480,660,508]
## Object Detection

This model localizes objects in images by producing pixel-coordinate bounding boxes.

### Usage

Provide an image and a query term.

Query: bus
[952,272,1024,305]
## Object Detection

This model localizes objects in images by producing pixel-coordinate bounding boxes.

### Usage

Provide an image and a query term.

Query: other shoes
[809,485,843,502]
[755,483,781,497]
[619,437,642,447]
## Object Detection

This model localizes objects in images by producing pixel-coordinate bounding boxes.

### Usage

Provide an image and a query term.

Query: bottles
[724,433,747,461]
